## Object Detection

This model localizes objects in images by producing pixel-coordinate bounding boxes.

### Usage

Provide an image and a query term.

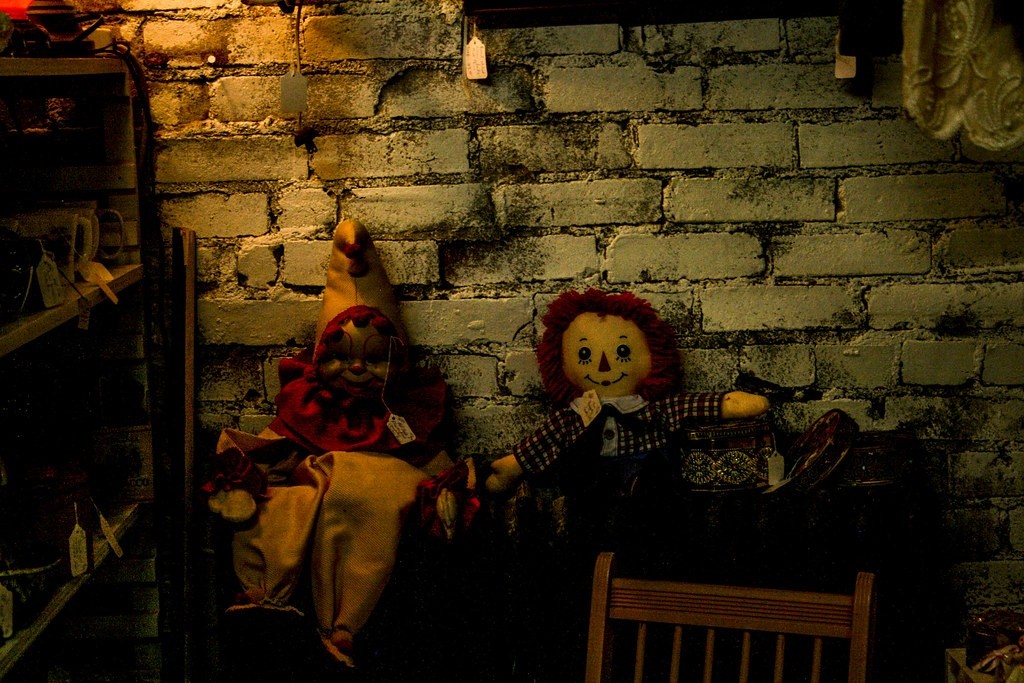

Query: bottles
[35,470,96,577]
[2,230,33,322]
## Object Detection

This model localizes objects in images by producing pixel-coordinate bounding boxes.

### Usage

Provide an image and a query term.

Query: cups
[10,198,126,293]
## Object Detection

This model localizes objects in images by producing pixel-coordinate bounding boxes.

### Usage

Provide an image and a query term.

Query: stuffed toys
[200,217,455,672]
[482,287,770,576]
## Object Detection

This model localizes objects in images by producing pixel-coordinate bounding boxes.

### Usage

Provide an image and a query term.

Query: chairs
[585,550,874,683]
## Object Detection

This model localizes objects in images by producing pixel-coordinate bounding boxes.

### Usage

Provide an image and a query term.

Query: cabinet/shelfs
[0,43,172,677]
